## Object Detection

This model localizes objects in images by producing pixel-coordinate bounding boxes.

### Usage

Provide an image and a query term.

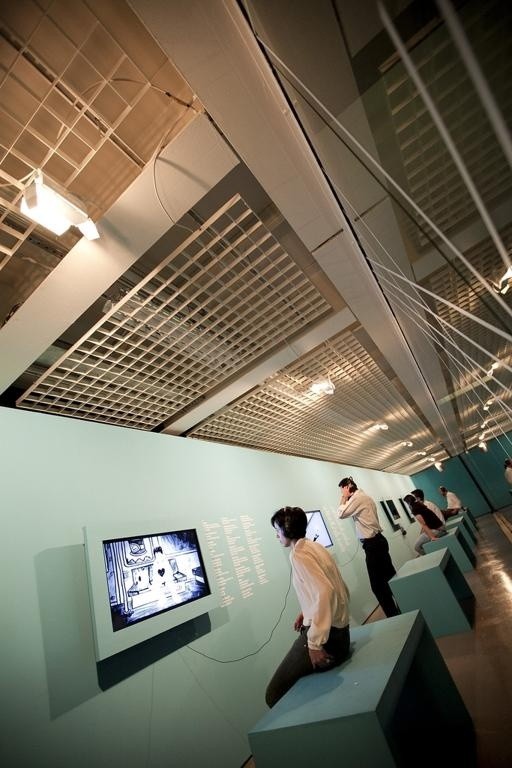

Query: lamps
[20,168,100,246]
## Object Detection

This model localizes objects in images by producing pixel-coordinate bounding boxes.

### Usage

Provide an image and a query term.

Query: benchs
[386,547,475,637]
[421,507,476,573]
[249,609,474,767]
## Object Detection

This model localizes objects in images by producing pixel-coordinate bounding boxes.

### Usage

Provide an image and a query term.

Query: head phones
[281,507,296,539]
[346,476,353,493]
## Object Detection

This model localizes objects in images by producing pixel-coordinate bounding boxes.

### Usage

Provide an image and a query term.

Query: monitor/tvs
[401,497,415,518]
[81,516,223,663]
[304,510,334,548]
[383,499,402,525]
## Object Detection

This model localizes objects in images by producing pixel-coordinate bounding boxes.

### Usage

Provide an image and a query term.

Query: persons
[266,508,351,711]
[403,495,446,557]
[337,477,401,619]
[503,457,512,485]
[149,546,174,589]
[439,486,462,516]
[410,489,445,533]
